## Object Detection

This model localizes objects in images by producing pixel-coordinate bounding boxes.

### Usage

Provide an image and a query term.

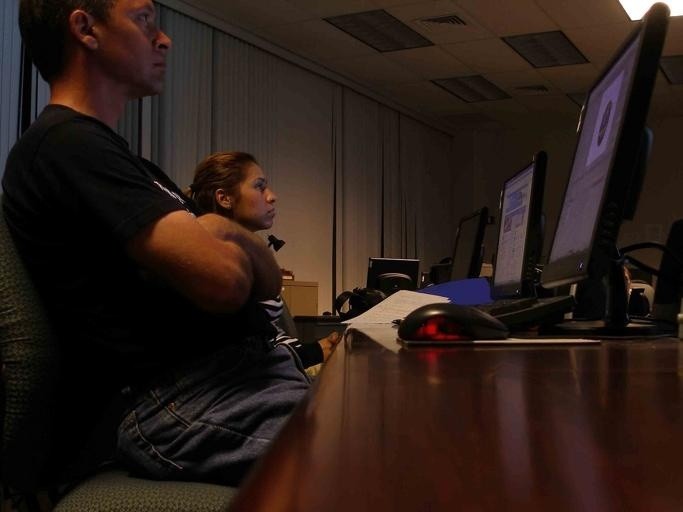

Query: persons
[2,0,315,492]
[174,148,341,369]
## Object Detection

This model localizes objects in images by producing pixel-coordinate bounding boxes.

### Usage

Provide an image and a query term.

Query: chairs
[0,220,237,512]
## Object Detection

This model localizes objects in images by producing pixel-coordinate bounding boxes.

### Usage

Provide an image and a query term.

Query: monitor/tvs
[489,148,548,298]
[447,205,490,281]
[540,3,674,339]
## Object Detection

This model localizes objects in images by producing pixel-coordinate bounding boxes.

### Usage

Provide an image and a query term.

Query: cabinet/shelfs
[281,280,319,316]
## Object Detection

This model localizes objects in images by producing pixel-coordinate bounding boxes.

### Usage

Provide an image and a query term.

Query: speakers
[366,256,421,296]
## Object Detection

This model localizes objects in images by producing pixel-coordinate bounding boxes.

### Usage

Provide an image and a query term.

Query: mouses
[396,302,509,340]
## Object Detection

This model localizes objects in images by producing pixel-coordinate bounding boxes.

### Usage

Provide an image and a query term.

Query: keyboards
[467,296,574,326]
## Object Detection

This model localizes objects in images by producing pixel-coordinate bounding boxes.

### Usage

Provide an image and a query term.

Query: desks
[228,316,683,512]
